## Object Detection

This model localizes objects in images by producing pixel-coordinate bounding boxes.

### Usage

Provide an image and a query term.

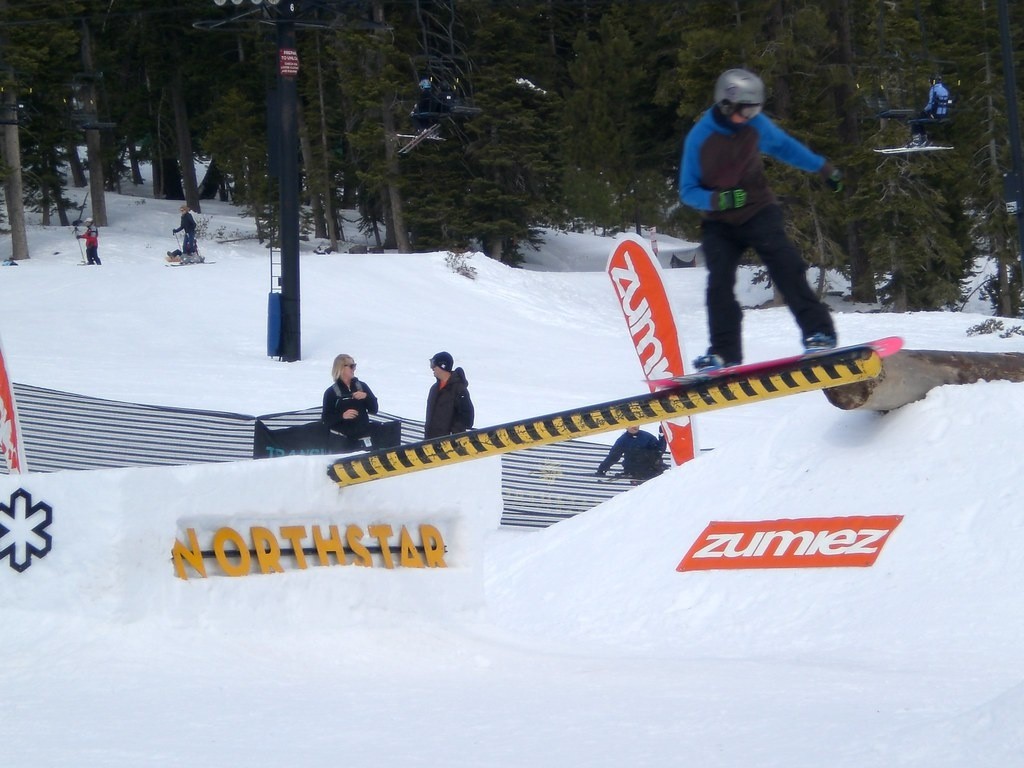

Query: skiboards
[164,260,217,268]
[76,259,88,266]
[872,145,955,154]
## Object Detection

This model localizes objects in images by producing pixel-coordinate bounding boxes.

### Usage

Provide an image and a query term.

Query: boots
[904,134,922,147]
[920,134,930,146]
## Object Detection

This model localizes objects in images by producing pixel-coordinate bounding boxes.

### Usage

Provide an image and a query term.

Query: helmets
[714,69,764,115]
[930,72,943,83]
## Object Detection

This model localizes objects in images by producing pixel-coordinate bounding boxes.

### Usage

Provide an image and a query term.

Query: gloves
[826,168,844,194]
[719,189,748,210]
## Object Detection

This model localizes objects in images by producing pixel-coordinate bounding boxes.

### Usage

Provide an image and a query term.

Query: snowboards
[164,256,182,262]
[639,336,905,390]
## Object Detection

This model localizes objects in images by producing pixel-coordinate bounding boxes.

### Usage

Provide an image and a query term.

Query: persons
[905,74,949,148]
[678,68,843,372]
[594,423,666,479]
[322,354,378,451]
[424,351,474,440]
[76,218,101,265]
[167,206,197,264]
[411,79,457,136]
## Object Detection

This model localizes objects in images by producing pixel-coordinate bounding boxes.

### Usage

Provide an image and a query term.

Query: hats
[429,351,454,371]
[84,218,93,222]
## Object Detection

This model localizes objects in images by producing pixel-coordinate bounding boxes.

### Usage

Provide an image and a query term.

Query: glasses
[344,364,356,370]
[736,103,763,119]
[431,360,435,369]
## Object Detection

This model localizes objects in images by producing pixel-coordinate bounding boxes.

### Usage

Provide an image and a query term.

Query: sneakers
[803,332,836,348]
[692,354,723,367]
